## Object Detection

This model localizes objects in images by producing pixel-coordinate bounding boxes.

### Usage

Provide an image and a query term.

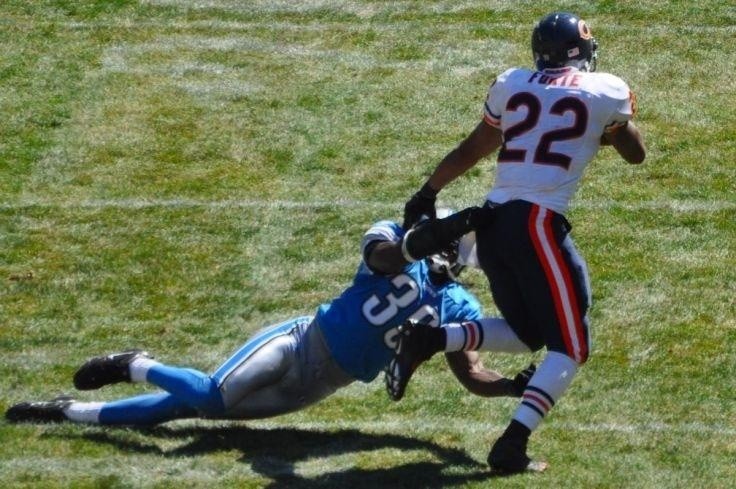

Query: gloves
[511,362,536,397]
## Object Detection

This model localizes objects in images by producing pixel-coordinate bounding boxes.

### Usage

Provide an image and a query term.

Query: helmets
[530,12,601,73]
[415,208,468,278]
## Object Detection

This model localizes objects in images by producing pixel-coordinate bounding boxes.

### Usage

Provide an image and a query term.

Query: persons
[4,204,538,425]
[384,10,648,472]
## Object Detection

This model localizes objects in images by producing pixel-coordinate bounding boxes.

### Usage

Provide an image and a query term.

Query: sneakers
[384,317,432,403]
[72,349,154,392]
[402,182,440,234]
[485,443,549,474]
[4,393,78,424]
